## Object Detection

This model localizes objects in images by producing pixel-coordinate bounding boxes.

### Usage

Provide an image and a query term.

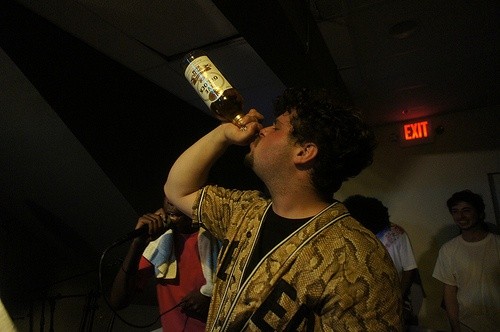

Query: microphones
[108,214,172,248]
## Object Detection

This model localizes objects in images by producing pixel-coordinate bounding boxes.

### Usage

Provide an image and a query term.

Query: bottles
[180,47,260,140]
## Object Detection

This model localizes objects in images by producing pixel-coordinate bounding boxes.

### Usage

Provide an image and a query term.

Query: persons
[109,92,423,332]
[431,190,500,332]
[0,298,16,331]
